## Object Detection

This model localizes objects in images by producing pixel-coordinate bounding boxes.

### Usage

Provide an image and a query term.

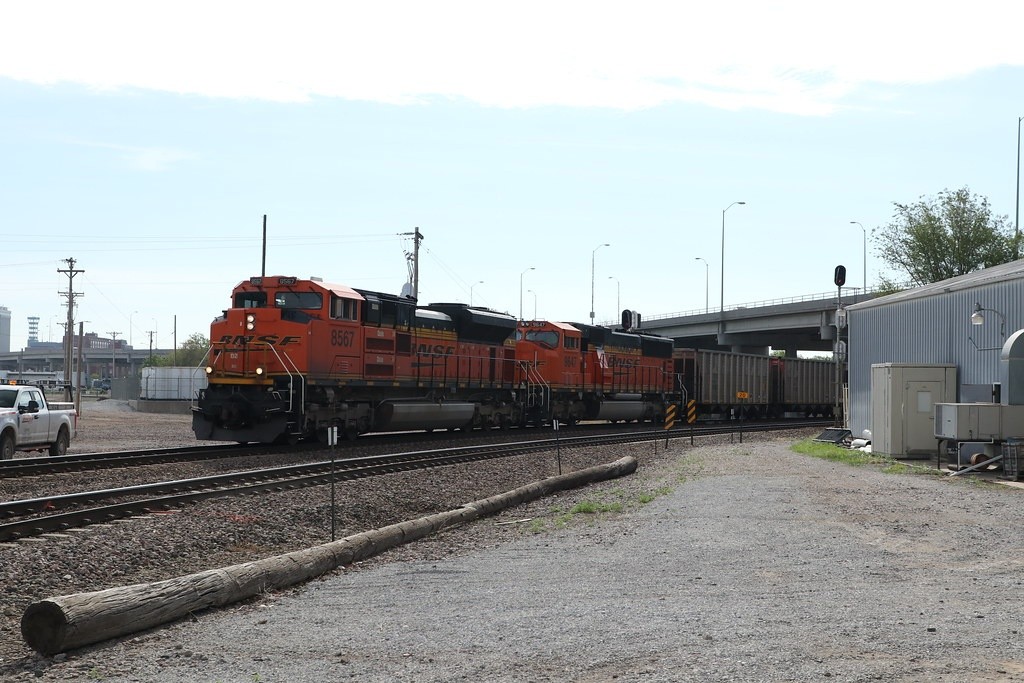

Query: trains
[189,275,847,444]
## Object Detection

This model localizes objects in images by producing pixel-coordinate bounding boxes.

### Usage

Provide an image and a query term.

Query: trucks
[0,370,120,391]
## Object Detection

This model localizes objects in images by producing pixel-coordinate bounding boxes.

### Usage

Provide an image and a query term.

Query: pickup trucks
[0,385,77,460]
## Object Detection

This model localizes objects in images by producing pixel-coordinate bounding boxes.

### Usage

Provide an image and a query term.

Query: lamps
[971,303,1005,336]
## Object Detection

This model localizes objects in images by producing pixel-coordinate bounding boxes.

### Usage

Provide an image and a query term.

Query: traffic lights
[834,265,846,286]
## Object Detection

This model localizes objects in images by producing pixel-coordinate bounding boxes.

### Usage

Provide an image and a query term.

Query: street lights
[520,268,535,319]
[850,221,866,294]
[152,318,157,348]
[592,243,610,324]
[528,290,537,320]
[471,281,484,306]
[720,201,745,311]
[609,277,620,324]
[695,257,708,313]
[129,311,137,345]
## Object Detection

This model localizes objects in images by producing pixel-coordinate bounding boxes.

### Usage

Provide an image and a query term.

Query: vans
[27,379,76,391]
